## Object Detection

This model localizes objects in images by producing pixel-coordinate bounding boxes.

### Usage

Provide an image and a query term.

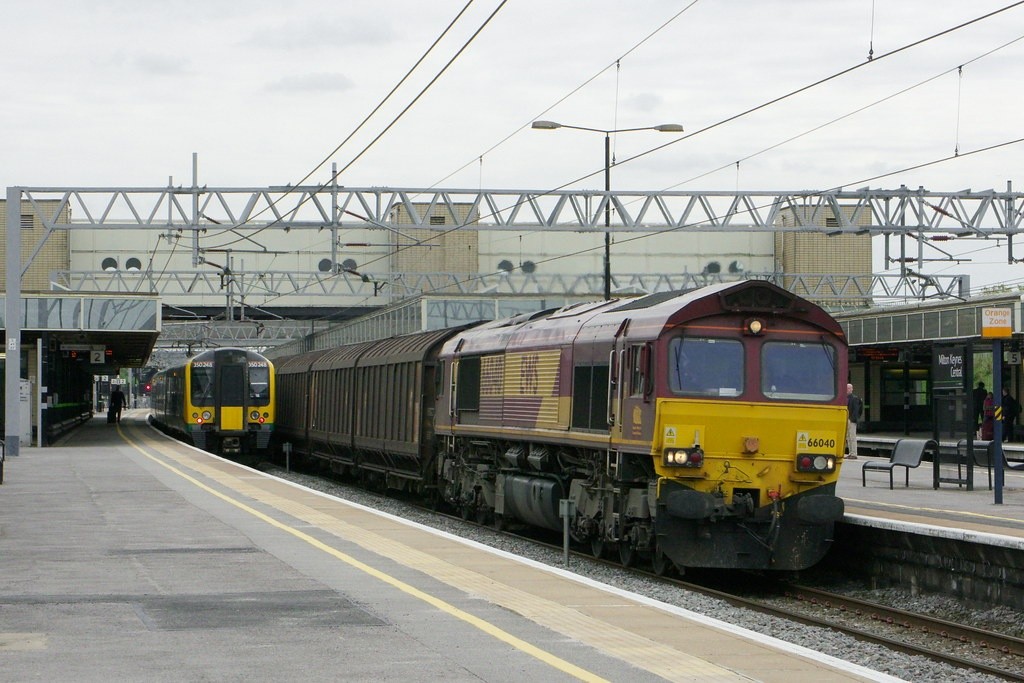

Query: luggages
[107,405,115,423]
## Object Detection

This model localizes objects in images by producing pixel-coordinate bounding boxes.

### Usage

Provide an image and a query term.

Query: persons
[972,382,988,434]
[1001,387,1016,443]
[983,392,994,423]
[844,383,859,459]
[110,385,127,424]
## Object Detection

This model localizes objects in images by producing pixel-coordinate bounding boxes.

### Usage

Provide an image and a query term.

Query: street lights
[531,121,685,301]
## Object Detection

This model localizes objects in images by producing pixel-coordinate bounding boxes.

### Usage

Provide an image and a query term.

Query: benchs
[862,439,938,489]
[957,440,1024,490]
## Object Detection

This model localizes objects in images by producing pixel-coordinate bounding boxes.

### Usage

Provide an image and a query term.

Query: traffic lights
[146,384,150,392]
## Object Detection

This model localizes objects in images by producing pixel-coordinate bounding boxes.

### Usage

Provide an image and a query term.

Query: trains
[149,347,276,471]
[272,279,848,588]
